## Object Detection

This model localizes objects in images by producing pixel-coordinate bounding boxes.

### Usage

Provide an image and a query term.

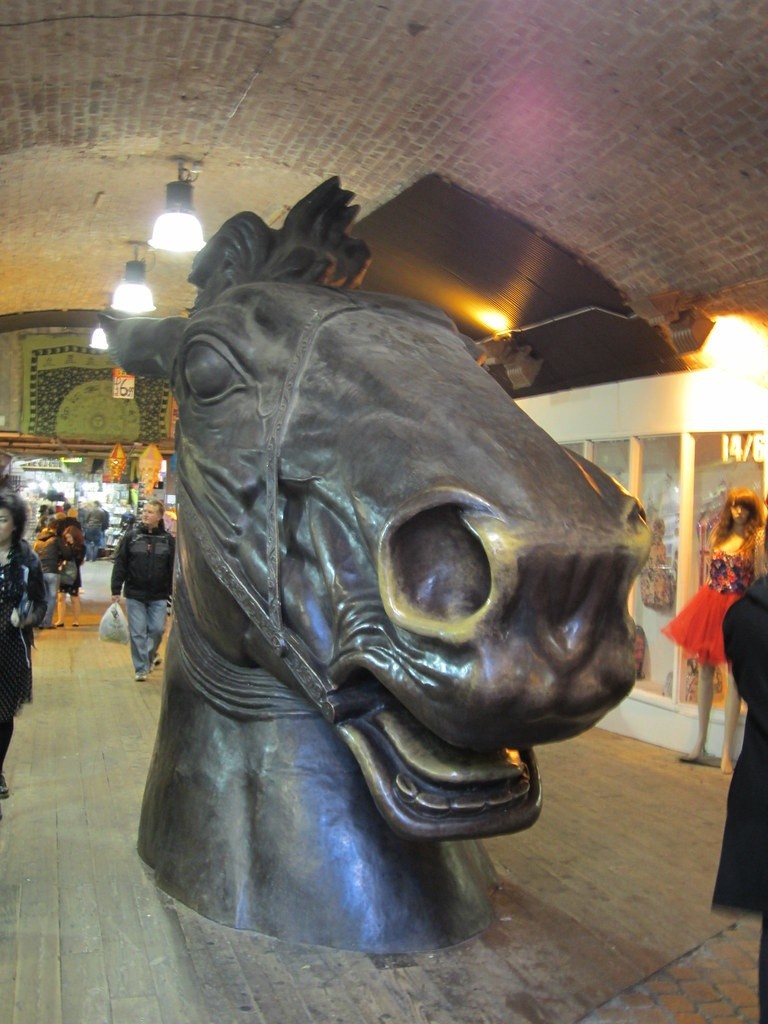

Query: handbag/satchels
[59,559,77,587]
[98,603,130,645]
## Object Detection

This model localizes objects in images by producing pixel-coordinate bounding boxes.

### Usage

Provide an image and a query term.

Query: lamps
[110,259,157,313]
[503,350,541,390]
[668,307,716,356]
[88,317,109,349]
[147,181,206,252]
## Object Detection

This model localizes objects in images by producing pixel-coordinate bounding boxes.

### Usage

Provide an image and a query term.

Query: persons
[0,491,49,800]
[108,504,135,561]
[34,517,75,630]
[661,488,768,772]
[112,499,176,681]
[52,526,87,627]
[712,512,768,1024]
[86,501,107,563]
[34,501,109,546]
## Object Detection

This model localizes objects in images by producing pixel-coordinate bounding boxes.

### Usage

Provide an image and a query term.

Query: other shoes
[149,663,155,672]
[57,623,64,627]
[46,625,56,629]
[135,674,147,681]
[72,622,80,627]
[0,775,9,798]
